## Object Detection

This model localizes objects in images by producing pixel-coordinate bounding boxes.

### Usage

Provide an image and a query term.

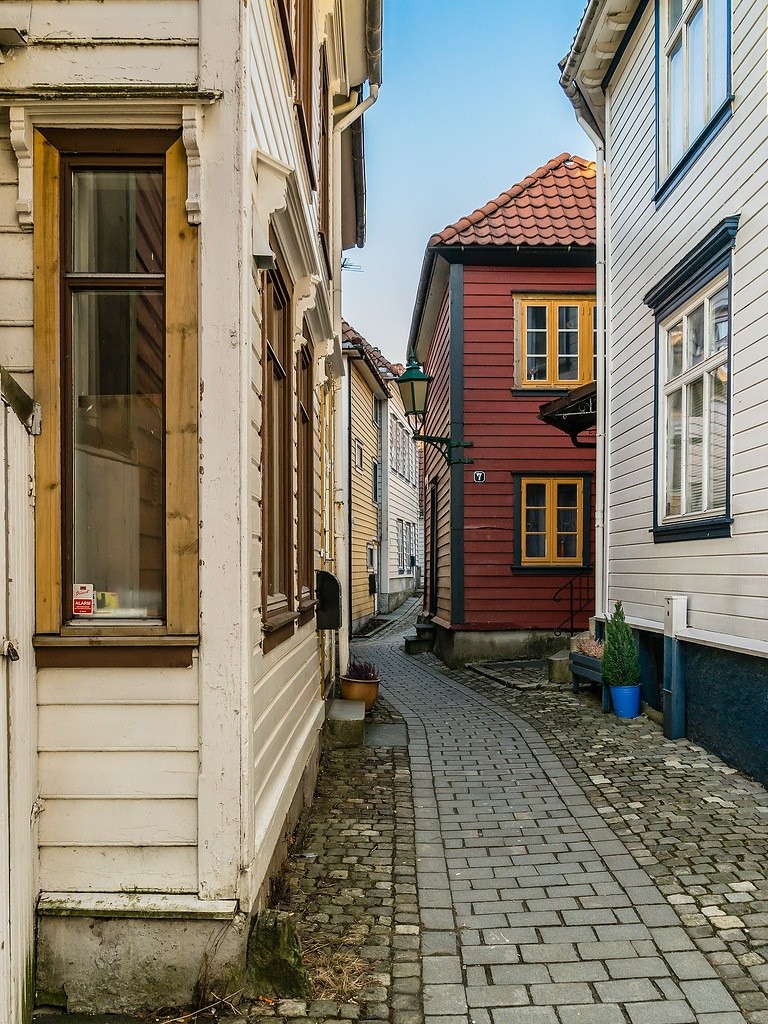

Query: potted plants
[338,651,382,713]
[601,600,642,719]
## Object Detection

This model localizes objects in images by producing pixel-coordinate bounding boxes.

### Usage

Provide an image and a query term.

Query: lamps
[395,344,451,466]
